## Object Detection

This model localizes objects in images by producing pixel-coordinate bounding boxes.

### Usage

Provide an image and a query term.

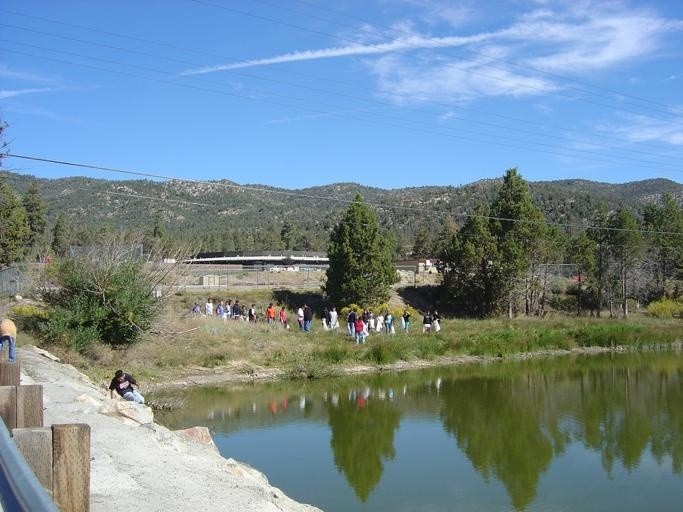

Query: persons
[205,376,447,424]
[0,316,18,365]
[188,295,442,346]
[107,369,147,405]
